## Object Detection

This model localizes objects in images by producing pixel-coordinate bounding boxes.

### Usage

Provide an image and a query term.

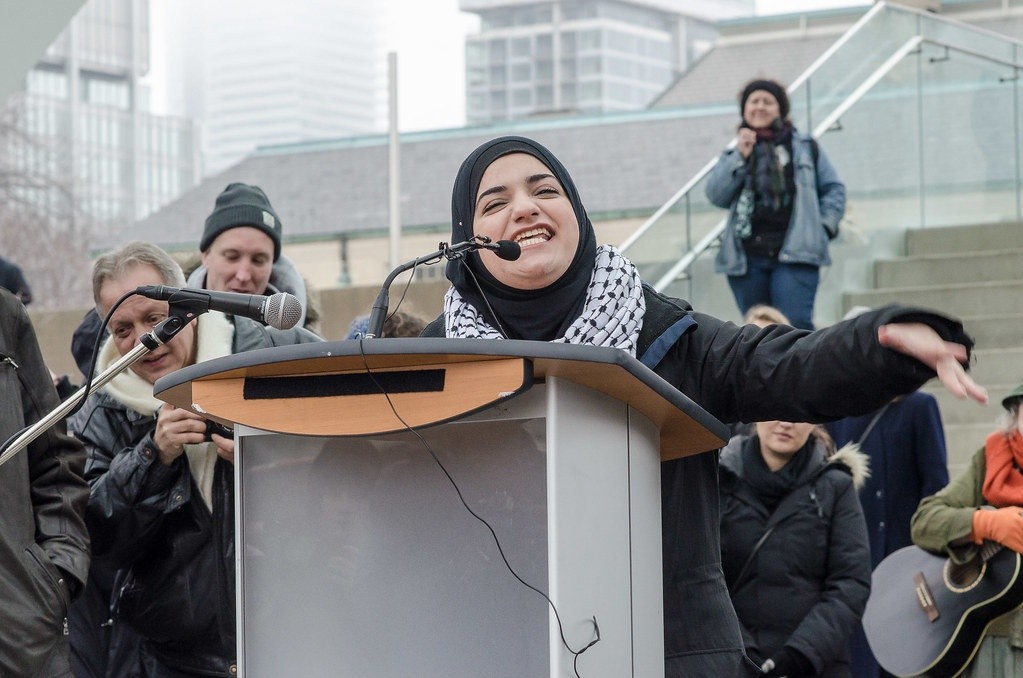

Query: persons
[706,72,846,330]
[1,180,1023,678]
[424,135,988,678]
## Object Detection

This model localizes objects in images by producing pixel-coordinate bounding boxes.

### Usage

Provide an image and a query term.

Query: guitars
[862,519,1023,678]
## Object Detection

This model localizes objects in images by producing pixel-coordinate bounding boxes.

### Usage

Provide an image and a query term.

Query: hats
[1001,384,1023,411]
[200,183,282,264]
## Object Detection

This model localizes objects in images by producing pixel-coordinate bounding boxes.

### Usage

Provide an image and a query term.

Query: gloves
[971,506,1023,555]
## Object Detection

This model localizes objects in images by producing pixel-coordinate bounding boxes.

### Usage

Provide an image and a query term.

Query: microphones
[137,284,302,329]
[365,239,522,339]
[477,235,492,244]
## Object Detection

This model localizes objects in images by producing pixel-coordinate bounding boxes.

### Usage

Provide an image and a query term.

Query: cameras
[187,418,235,444]
